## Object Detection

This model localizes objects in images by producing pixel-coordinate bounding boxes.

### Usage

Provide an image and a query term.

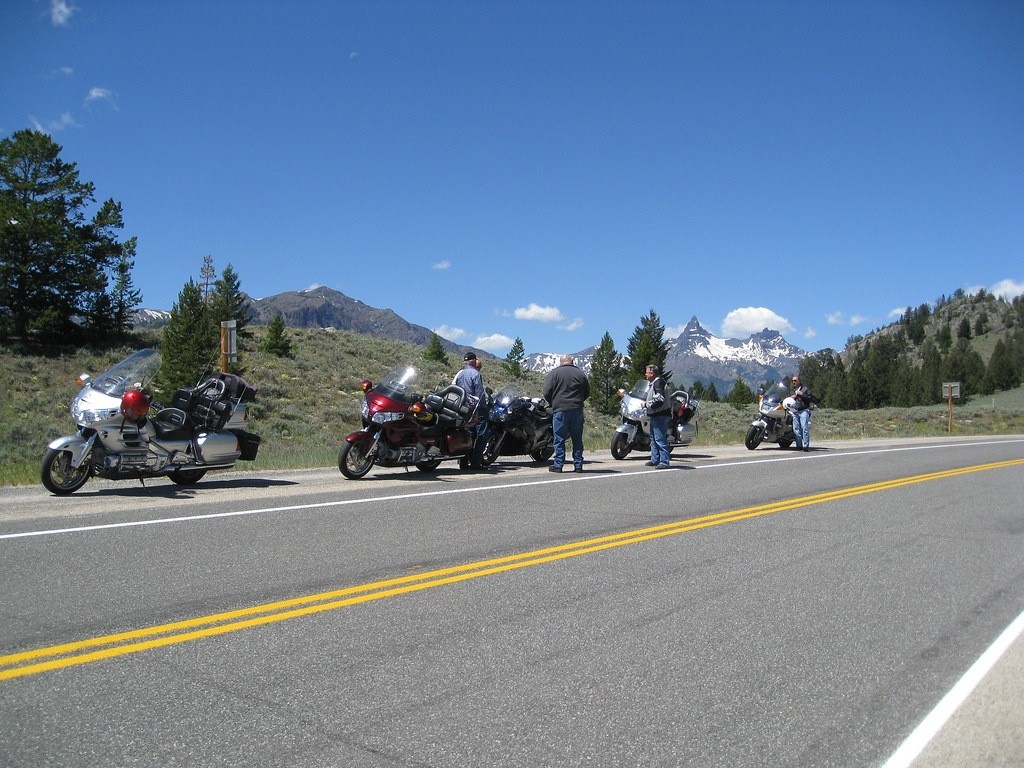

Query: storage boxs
[192,430,242,465]
[675,423,696,443]
[227,428,261,461]
[444,428,473,456]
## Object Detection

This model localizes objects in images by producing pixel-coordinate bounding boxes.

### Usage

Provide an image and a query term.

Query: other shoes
[575,468,582,473]
[804,449,808,452]
[795,448,803,451]
[655,464,668,469]
[644,461,655,466]
[549,466,562,473]
[460,457,490,473]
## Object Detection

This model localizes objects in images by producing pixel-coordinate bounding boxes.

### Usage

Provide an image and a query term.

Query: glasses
[792,380,796,382]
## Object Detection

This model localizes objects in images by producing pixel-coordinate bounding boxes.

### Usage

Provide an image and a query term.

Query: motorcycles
[610,379,701,462]
[336,365,484,482]
[39,348,262,495]
[744,381,812,450]
[480,383,572,468]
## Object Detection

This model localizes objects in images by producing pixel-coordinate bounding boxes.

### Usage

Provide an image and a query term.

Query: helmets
[783,397,798,413]
[121,390,149,424]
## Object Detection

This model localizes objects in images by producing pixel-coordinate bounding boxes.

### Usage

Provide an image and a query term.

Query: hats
[464,352,478,361]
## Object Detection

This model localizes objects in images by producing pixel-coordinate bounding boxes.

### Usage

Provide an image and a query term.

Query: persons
[543,355,590,473]
[790,376,812,452]
[641,365,671,469]
[453,352,489,472]
